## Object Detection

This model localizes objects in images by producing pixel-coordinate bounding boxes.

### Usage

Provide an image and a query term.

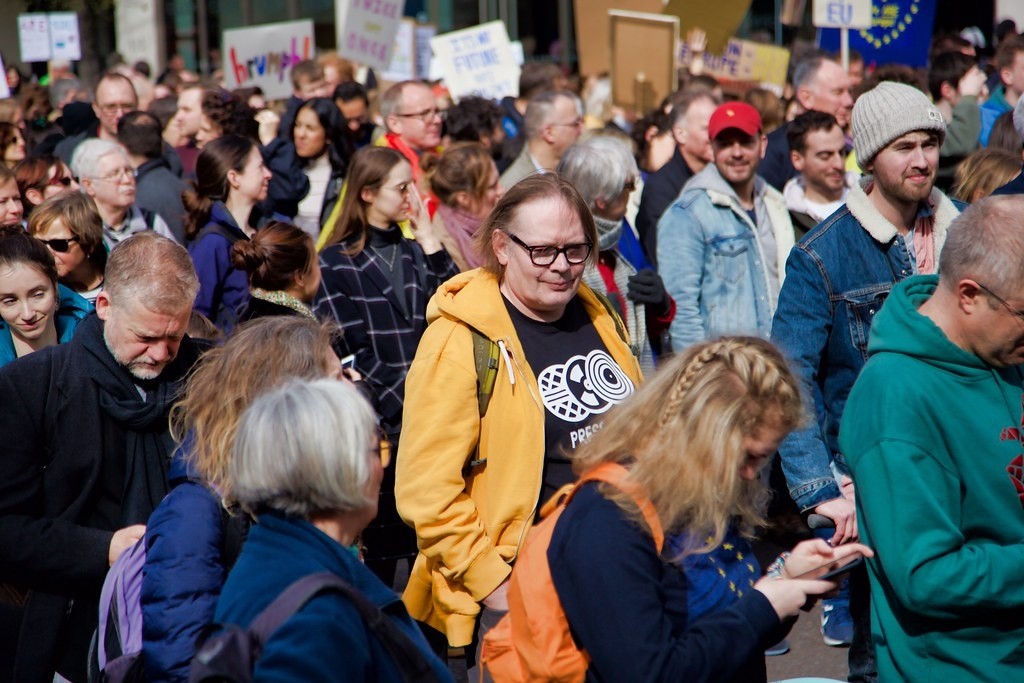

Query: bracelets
[767,552,789,578]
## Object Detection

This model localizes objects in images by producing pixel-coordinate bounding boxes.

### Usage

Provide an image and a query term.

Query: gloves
[628,270,671,319]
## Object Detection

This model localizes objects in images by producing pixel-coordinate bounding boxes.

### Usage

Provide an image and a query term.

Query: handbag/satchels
[170,559,420,683]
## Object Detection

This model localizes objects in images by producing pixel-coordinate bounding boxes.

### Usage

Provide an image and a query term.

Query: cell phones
[791,552,863,580]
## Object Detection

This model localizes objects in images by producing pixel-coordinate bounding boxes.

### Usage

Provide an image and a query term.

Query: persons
[0,20,1024,683]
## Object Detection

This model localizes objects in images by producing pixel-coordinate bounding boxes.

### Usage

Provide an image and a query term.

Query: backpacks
[478,461,663,683]
[85,478,259,683]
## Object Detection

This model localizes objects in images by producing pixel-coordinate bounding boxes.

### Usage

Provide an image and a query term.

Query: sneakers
[821,592,852,645]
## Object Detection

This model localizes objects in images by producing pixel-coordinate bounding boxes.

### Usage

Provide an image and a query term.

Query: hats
[709,101,764,143]
[850,81,946,175]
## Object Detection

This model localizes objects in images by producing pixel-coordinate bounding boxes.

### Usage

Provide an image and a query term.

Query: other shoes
[764,641,791,655]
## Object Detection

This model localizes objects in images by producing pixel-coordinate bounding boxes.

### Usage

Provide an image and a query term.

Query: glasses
[503,227,595,266]
[96,100,137,115]
[550,117,580,129]
[618,176,636,191]
[88,166,139,183]
[7,135,23,143]
[374,180,417,197]
[37,176,80,187]
[39,236,81,253]
[398,107,445,123]
